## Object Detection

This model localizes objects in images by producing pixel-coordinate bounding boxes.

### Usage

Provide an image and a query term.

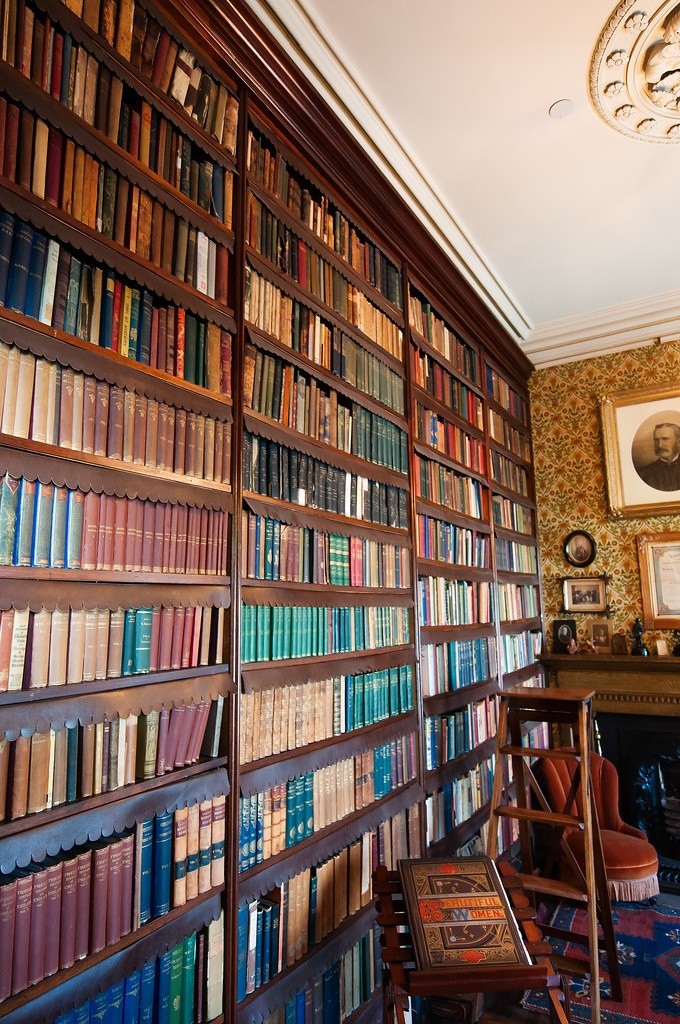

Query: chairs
[531,746,661,907]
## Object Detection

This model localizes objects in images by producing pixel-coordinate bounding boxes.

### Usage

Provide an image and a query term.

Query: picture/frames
[563,530,597,568]
[586,619,613,654]
[397,854,529,972]
[556,572,615,618]
[552,620,577,655]
[599,382,680,521]
[635,530,680,630]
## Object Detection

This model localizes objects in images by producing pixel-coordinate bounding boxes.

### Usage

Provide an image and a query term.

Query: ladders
[477,682,624,1024]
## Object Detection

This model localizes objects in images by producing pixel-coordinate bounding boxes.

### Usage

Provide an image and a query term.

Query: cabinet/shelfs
[0,0,552,1024]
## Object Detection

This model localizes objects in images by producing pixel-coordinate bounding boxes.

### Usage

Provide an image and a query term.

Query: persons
[575,589,597,603]
[575,537,587,561]
[637,423,680,491]
[560,627,569,641]
[632,618,644,646]
[596,630,606,646]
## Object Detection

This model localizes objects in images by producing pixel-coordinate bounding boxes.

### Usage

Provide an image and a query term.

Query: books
[0,0,547,1024]
